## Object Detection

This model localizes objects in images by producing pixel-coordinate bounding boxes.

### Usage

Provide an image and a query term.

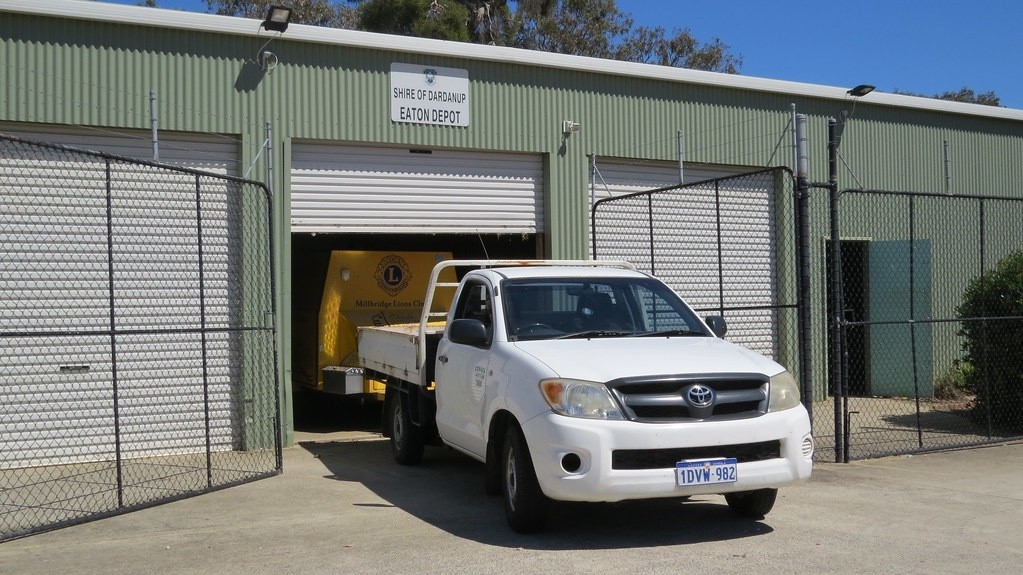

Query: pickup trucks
[357,259,814,533]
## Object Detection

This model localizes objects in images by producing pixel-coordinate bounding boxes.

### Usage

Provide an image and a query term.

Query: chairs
[577,290,613,325]
